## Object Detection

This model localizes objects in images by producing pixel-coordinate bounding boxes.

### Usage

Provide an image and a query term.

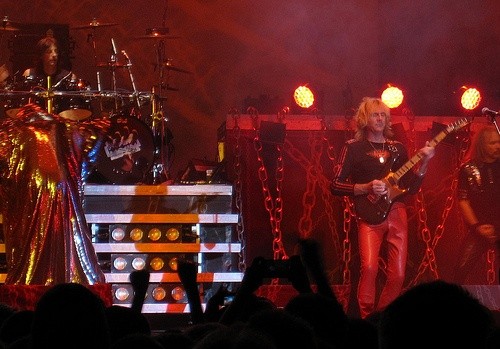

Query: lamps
[378,83,409,112]
[451,83,483,116]
[292,80,322,112]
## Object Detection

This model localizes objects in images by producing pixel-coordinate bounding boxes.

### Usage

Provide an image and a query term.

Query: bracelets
[472,223,481,230]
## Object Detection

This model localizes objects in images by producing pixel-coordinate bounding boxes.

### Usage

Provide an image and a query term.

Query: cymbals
[96,62,131,67]
[130,32,177,39]
[70,23,120,29]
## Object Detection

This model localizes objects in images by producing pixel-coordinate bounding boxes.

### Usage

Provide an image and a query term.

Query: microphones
[121,50,131,63]
[482,107,500,116]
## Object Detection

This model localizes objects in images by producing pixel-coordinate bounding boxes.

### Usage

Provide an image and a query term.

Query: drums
[54,79,93,120]
[132,91,164,125]
[4,74,44,118]
[82,113,154,184]
[91,88,133,117]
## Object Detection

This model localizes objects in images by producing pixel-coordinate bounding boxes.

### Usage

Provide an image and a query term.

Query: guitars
[353,117,468,224]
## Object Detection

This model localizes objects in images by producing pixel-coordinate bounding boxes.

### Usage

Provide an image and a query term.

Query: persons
[0,240,500,349]
[331,95,445,319]
[446,123,500,300]
[22,35,81,85]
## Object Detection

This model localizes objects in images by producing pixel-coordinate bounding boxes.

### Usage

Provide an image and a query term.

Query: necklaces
[369,142,385,162]
